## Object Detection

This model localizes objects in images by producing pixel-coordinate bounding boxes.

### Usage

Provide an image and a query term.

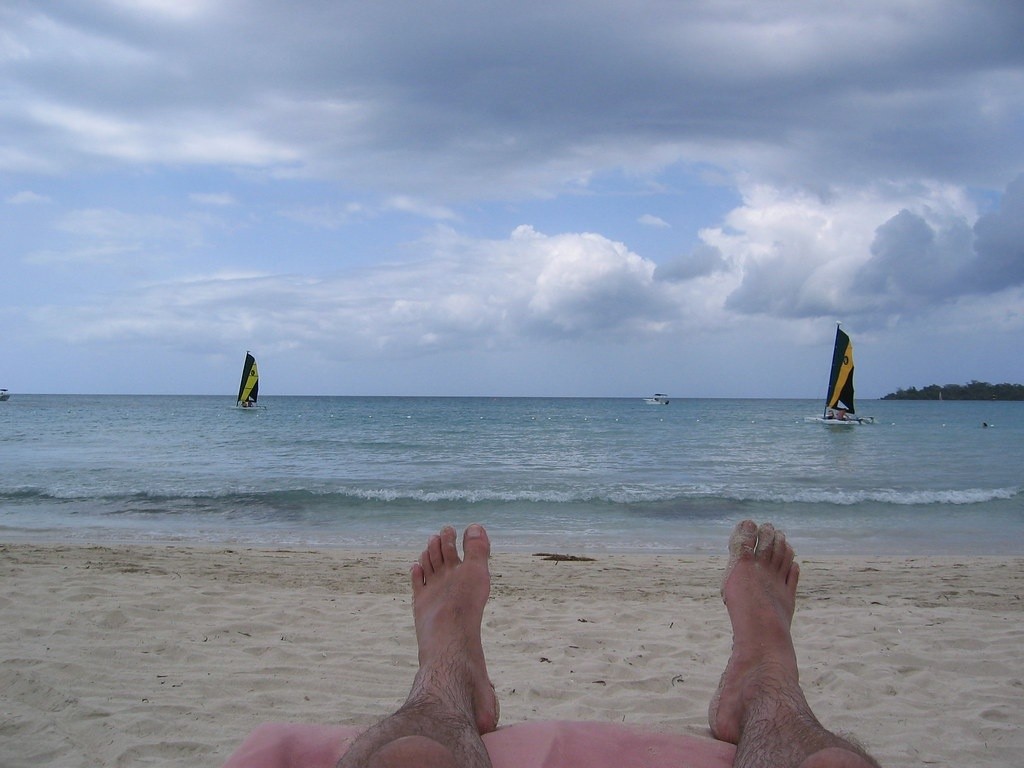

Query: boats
[644,394,670,406]
[0,388,10,401]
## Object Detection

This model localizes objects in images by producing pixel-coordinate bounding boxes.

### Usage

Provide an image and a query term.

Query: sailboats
[228,350,269,411]
[804,321,882,425]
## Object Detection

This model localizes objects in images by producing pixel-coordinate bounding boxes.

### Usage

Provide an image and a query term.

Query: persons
[336,520,881,768]
[825,410,834,420]
[836,408,850,421]
